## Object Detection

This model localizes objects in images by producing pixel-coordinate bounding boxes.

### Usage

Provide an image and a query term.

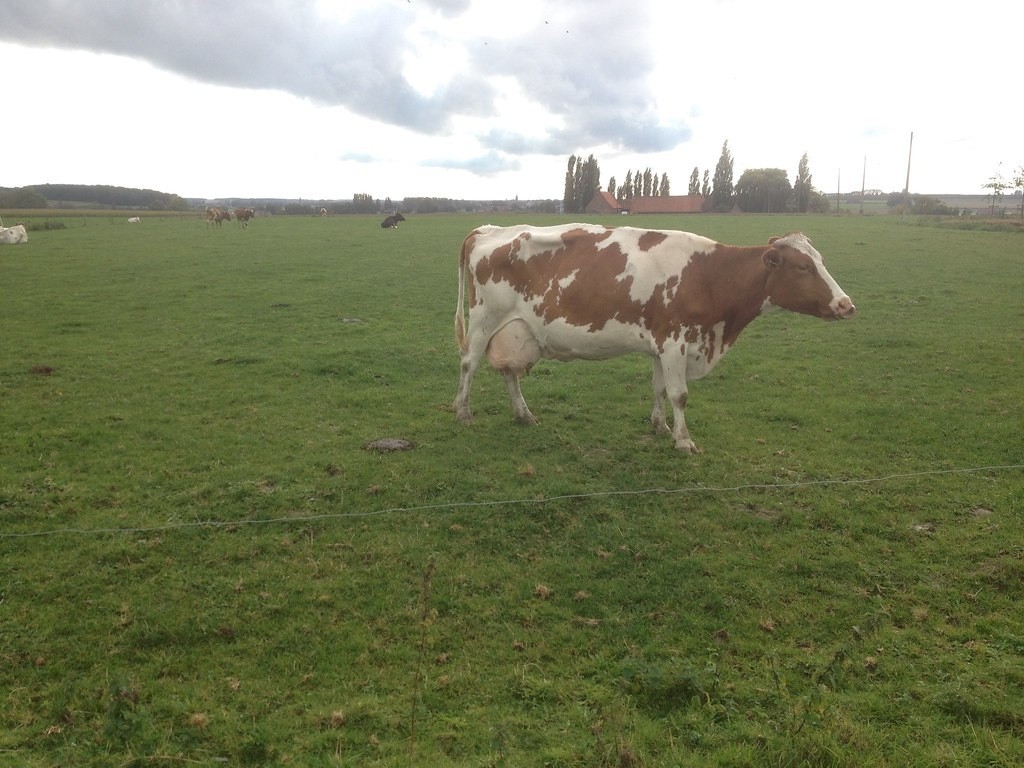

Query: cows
[380,209,405,229]
[453,220,857,453]
[319,207,329,217]
[205,206,256,233]
[128,215,141,224]
[0,225,28,244]
